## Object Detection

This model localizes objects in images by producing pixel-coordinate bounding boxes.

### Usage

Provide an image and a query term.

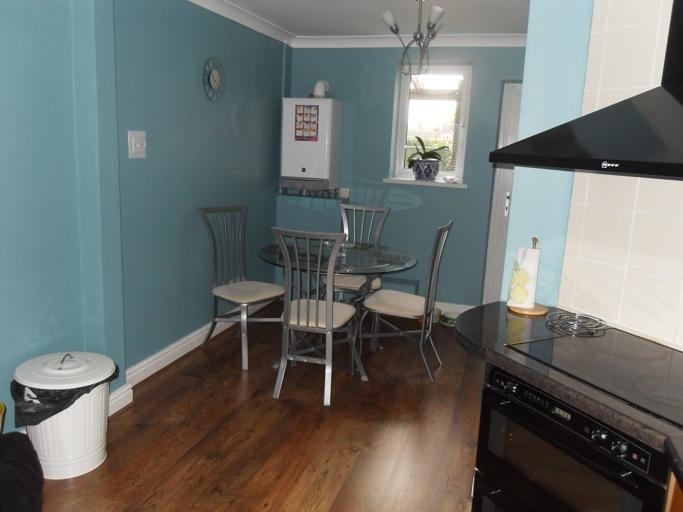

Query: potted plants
[406,135,449,181]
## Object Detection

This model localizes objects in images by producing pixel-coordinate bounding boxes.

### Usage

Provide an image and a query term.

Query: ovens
[471,361,670,512]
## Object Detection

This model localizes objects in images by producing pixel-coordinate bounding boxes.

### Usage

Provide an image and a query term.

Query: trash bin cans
[5,352,118,479]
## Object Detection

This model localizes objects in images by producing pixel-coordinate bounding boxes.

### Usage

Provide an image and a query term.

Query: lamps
[382,0,445,78]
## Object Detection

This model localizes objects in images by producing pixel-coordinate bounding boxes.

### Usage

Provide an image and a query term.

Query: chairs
[196,203,299,372]
[320,203,391,352]
[270,226,368,407]
[357,219,454,383]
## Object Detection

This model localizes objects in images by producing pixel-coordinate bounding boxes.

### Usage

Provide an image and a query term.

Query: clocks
[202,56,227,103]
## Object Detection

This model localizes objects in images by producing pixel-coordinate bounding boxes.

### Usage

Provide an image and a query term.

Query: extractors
[488,0,683,181]
